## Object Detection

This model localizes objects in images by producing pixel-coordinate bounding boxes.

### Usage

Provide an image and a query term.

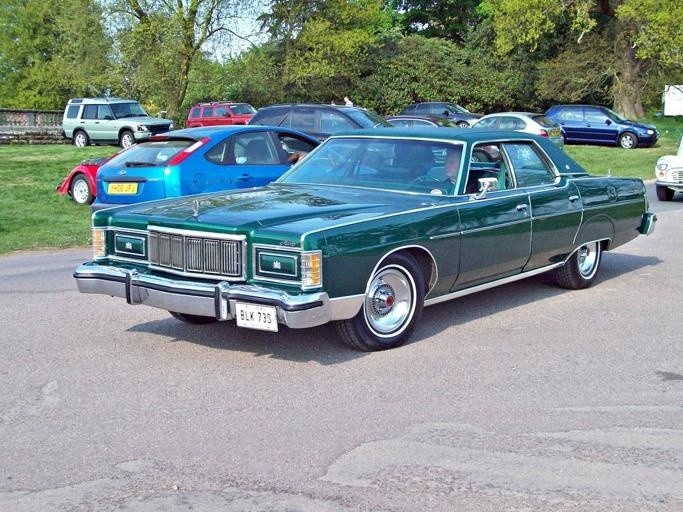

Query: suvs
[60,93,173,148]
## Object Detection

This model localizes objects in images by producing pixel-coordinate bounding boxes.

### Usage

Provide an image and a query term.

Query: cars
[91,124,376,217]
[649,135,682,202]
[69,125,657,352]
[56,154,115,207]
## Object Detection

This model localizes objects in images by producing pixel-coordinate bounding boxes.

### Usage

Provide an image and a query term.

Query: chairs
[415,163,445,184]
[377,167,409,180]
[467,168,499,195]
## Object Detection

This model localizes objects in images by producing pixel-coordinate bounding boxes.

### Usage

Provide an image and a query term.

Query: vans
[543,102,660,152]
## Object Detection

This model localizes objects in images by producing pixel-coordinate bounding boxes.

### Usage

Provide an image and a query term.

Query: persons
[283,150,306,163]
[427,149,480,196]
[343,95,353,107]
[330,99,337,106]
